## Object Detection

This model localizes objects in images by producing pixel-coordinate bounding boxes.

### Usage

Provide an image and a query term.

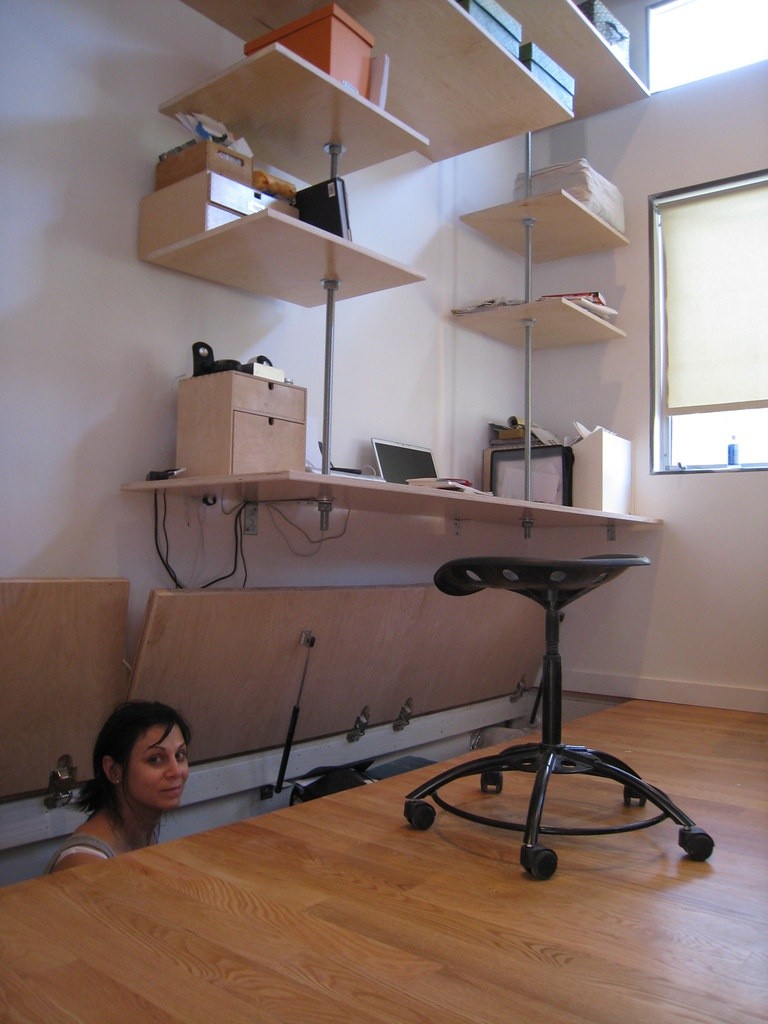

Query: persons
[43,698,194,875]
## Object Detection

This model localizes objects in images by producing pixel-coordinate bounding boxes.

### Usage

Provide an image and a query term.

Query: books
[535,291,618,321]
[405,478,494,498]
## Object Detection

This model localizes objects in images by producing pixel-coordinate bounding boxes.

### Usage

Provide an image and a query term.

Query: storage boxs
[174,370,308,477]
[457,0,522,60]
[155,141,253,190]
[519,42,576,112]
[577,0,630,68]
[243,2,376,101]
[137,169,299,262]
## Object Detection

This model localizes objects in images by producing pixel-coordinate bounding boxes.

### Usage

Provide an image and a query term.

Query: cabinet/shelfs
[122,0,664,540]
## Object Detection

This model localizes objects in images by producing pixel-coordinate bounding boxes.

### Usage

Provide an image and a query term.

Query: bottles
[727,435,738,468]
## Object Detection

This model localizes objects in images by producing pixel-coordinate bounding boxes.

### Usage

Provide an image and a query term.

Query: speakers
[193,341,216,378]
[247,355,272,367]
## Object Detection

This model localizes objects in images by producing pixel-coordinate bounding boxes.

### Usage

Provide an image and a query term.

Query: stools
[405,553,714,879]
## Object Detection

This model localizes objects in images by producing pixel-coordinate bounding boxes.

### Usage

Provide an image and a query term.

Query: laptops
[371,439,438,485]
